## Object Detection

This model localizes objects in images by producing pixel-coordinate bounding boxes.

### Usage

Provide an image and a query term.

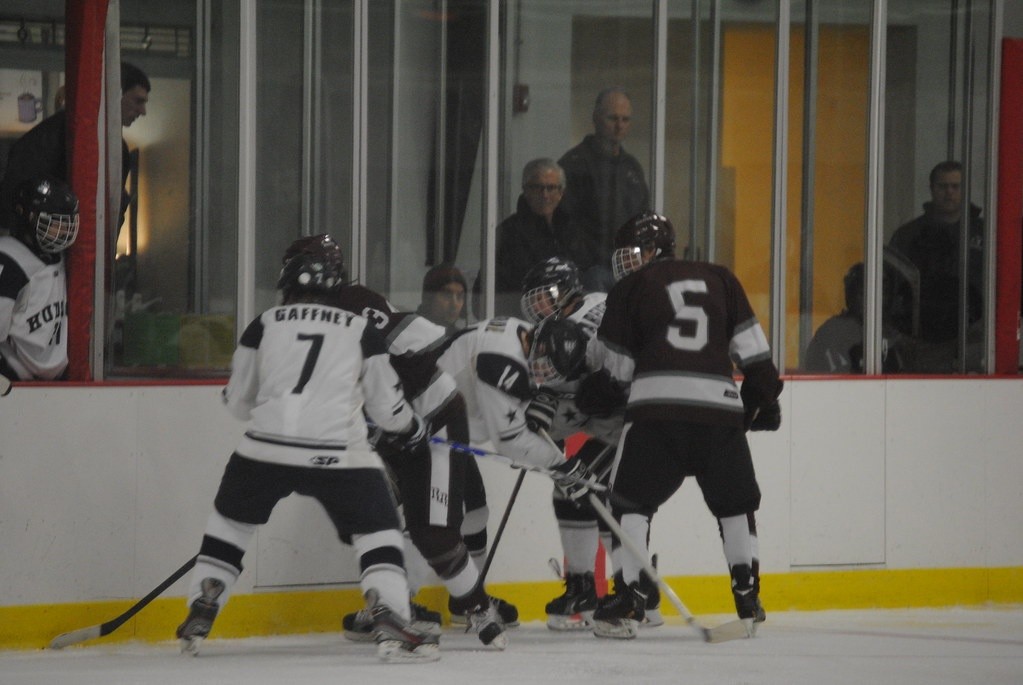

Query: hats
[423,261,470,320]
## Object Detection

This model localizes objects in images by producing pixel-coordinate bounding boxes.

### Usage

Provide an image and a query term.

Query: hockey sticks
[588,492,756,644]
[428,421,658,516]
[475,467,527,607]
[50,552,197,650]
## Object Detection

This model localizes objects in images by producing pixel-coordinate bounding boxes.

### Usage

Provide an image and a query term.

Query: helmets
[521,249,585,324]
[10,176,83,256]
[613,212,675,286]
[527,319,592,386]
[275,234,345,290]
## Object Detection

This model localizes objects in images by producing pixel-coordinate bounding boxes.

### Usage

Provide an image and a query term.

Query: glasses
[524,182,564,195]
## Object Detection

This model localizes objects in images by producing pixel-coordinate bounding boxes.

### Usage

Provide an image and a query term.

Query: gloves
[743,393,784,433]
[384,413,432,458]
[525,386,561,436]
[574,370,624,420]
[550,454,604,512]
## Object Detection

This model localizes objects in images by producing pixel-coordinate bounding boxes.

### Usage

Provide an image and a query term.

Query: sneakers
[634,554,664,626]
[447,587,520,630]
[728,558,766,636]
[464,591,510,652]
[340,597,444,644]
[372,604,443,663]
[545,556,600,632]
[591,568,645,639]
[176,594,220,658]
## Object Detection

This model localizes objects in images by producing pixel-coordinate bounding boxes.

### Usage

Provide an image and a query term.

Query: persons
[472,158,614,321]
[0,176,81,396]
[0,61,151,255]
[805,160,986,376]
[556,87,653,270]
[175,234,440,663]
[325,210,785,651]
